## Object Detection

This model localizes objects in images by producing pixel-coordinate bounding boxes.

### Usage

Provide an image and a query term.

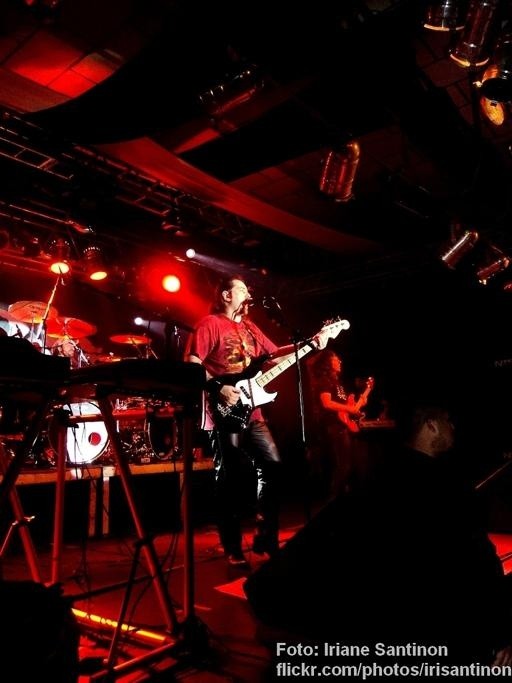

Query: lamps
[450,0,500,68]
[82,246,109,280]
[436,225,480,271]
[48,238,72,275]
[316,137,362,203]
[473,246,512,285]
[195,66,265,119]
[171,217,197,263]
[479,93,512,128]
[424,3,464,32]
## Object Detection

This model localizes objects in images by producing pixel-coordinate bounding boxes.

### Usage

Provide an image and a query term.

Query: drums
[115,399,179,460]
[35,399,110,468]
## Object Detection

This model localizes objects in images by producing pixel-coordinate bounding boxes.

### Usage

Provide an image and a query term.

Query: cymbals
[7,301,57,324]
[110,334,150,343]
[46,318,93,338]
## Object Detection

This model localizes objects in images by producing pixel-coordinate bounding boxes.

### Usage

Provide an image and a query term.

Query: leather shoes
[253,536,283,559]
[224,546,249,569]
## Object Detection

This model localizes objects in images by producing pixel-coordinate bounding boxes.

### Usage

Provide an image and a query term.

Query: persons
[183,273,322,569]
[308,345,370,476]
[50,335,77,357]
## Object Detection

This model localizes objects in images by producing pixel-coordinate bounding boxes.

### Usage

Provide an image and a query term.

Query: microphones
[248,295,274,307]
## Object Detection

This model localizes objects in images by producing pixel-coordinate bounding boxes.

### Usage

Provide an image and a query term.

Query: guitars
[339,376,376,432]
[205,319,351,434]
